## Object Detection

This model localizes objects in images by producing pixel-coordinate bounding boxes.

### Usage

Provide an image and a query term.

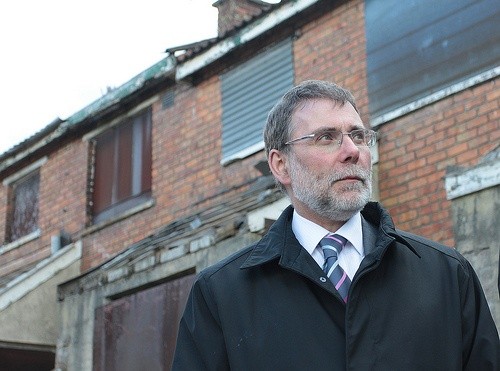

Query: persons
[170,80,500,371]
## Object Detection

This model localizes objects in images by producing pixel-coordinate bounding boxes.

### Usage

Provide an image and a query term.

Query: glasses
[285,127,379,148]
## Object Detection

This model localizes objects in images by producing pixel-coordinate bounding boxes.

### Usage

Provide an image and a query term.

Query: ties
[319,234,353,304]
